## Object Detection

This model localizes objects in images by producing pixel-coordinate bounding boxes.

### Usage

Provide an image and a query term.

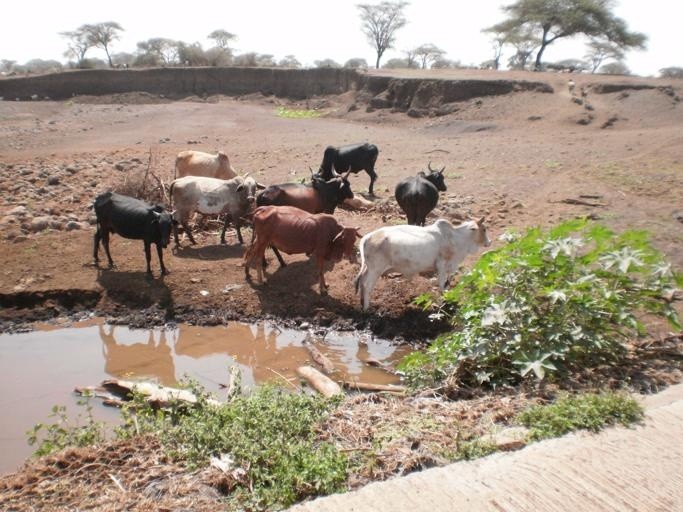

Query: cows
[241,205,363,297]
[251,162,355,268]
[174,150,250,180]
[168,175,266,248]
[395,160,448,227]
[308,142,379,197]
[91,191,177,283]
[353,212,493,312]
